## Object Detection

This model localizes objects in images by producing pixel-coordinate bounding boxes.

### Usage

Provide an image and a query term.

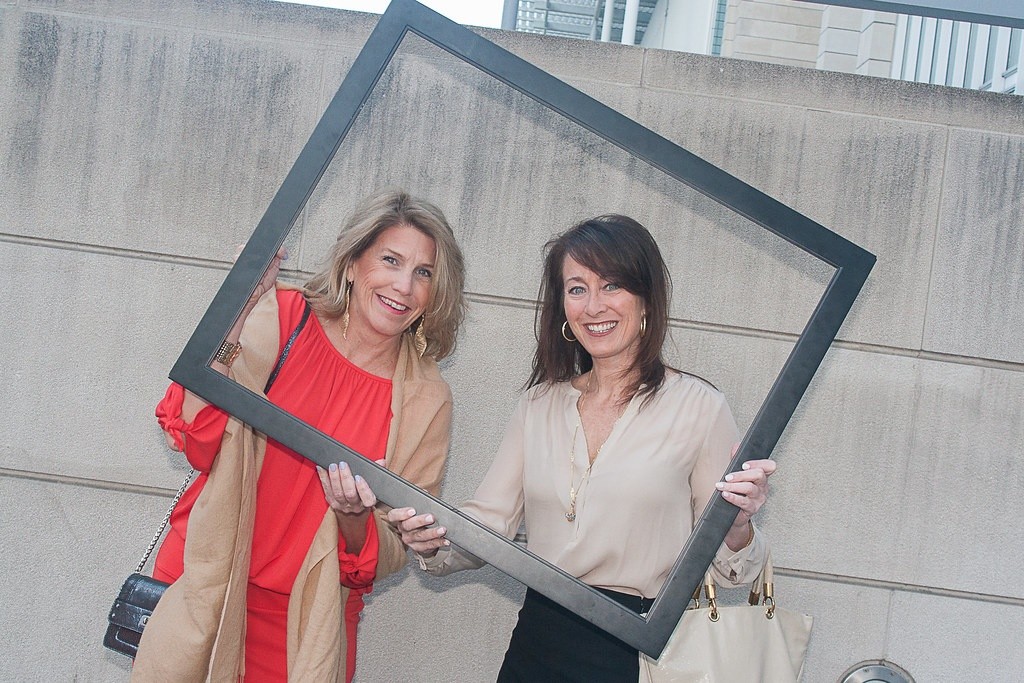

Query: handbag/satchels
[636,553,815,683]
[101,571,171,662]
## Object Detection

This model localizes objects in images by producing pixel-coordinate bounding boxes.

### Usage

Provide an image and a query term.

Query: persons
[386,213,777,683]
[131,190,465,683]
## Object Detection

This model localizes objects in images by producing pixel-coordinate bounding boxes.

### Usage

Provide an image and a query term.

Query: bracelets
[215,339,243,365]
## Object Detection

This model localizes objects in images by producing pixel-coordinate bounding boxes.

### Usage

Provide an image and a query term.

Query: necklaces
[566,366,620,520]
